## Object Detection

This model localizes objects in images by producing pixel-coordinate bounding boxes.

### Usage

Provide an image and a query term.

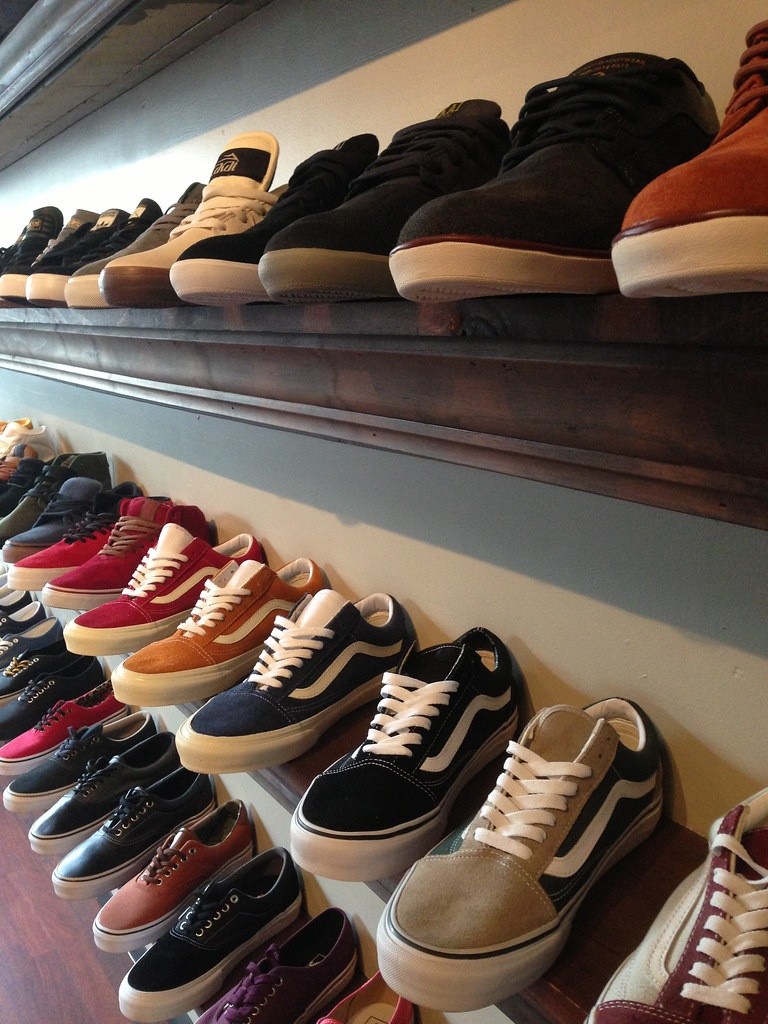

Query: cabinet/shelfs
[0,0,768,1024]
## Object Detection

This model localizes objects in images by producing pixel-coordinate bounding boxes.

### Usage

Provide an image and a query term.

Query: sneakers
[0,417,522,1024]
[0,33,768,305]
[369,693,668,1012]
[578,780,768,1024]
